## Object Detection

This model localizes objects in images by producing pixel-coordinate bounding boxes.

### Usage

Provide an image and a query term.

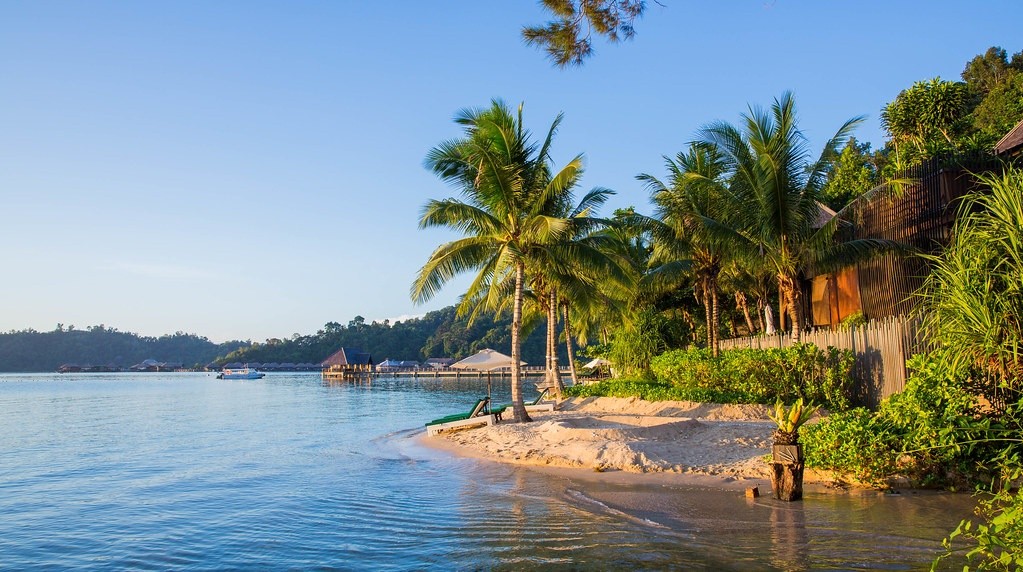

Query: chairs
[500,388,556,412]
[424,396,506,436]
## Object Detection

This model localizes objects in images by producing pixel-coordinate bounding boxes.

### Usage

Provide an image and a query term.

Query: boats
[217,362,266,380]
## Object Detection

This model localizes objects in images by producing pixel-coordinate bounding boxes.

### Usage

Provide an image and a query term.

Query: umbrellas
[582,357,617,378]
[449,348,528,415]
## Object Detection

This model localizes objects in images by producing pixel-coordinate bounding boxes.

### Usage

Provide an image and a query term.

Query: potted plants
[765,393,823,461]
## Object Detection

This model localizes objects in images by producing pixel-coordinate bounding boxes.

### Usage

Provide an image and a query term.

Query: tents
[58,360,328,371]
